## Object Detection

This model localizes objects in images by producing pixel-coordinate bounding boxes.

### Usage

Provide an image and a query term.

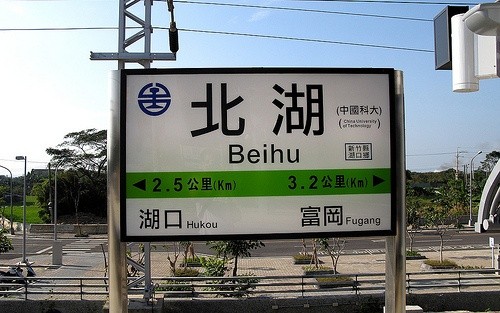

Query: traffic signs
[121,68,396,243]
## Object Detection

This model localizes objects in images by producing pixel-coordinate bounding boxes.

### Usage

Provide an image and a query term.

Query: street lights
[16,156,27,263]
[468,151,483,226]
